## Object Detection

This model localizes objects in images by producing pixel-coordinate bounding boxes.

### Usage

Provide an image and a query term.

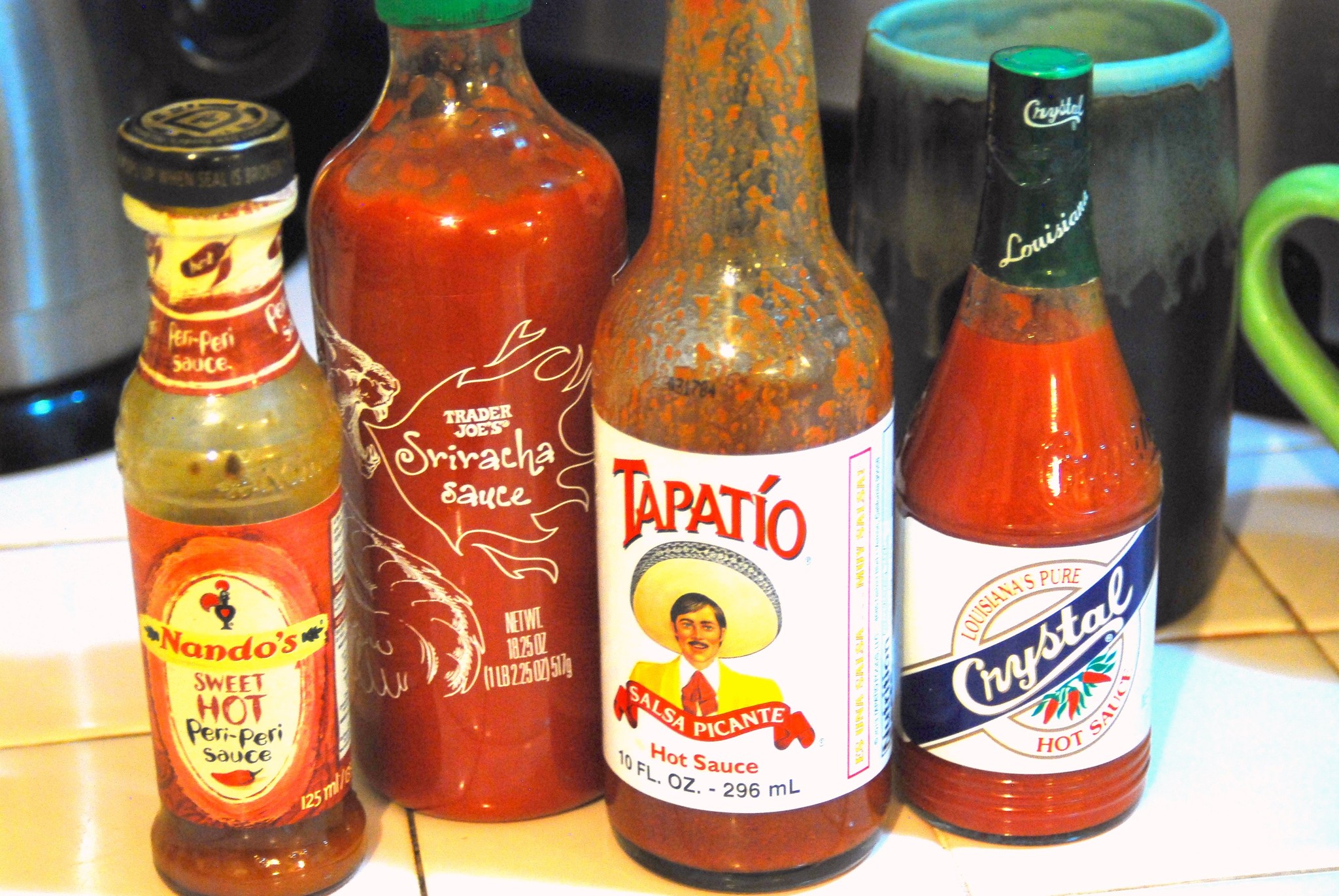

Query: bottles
[303,1,629,824]
[593,0,903,895]
[888,46,1165,847]
[115,98,370,896]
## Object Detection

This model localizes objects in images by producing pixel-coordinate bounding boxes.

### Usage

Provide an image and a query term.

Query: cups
[846,0,1240,626]
[3,2,173,391]
